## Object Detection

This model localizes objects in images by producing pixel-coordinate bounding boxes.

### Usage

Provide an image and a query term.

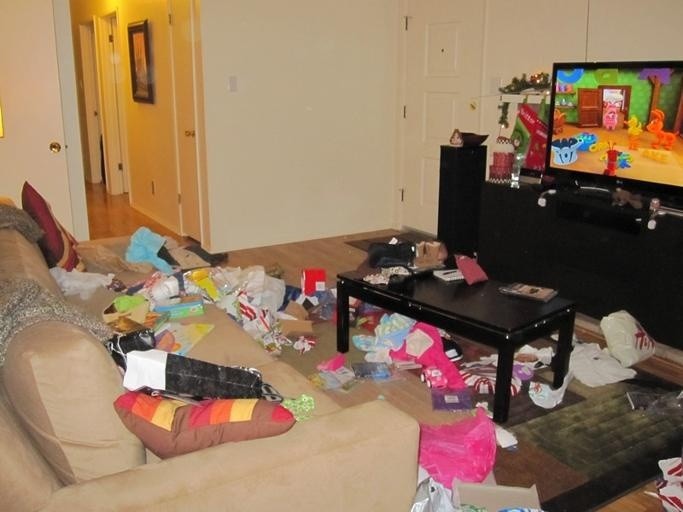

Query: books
[499,282,559,304]
[433,269,464,281]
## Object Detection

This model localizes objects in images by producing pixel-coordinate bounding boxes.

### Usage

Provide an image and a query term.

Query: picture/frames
[125,18,154,106]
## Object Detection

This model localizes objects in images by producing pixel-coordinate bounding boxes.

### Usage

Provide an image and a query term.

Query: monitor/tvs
[543,61,683,200]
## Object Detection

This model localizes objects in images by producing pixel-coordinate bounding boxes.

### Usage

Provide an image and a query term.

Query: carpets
[342,230,435,253]
[296,315,682,512]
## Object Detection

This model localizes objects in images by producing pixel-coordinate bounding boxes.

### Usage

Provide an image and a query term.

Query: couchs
[0,193,425,511]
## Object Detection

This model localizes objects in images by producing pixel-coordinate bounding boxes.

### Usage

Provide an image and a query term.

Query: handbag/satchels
[414,241,448,268]
[369,242,415,268]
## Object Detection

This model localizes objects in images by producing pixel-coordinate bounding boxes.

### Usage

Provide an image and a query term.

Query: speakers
[437,144,487,252]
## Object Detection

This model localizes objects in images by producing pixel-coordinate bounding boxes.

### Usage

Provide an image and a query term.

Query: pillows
[19,179,84,275]
[111,388,300,460]
